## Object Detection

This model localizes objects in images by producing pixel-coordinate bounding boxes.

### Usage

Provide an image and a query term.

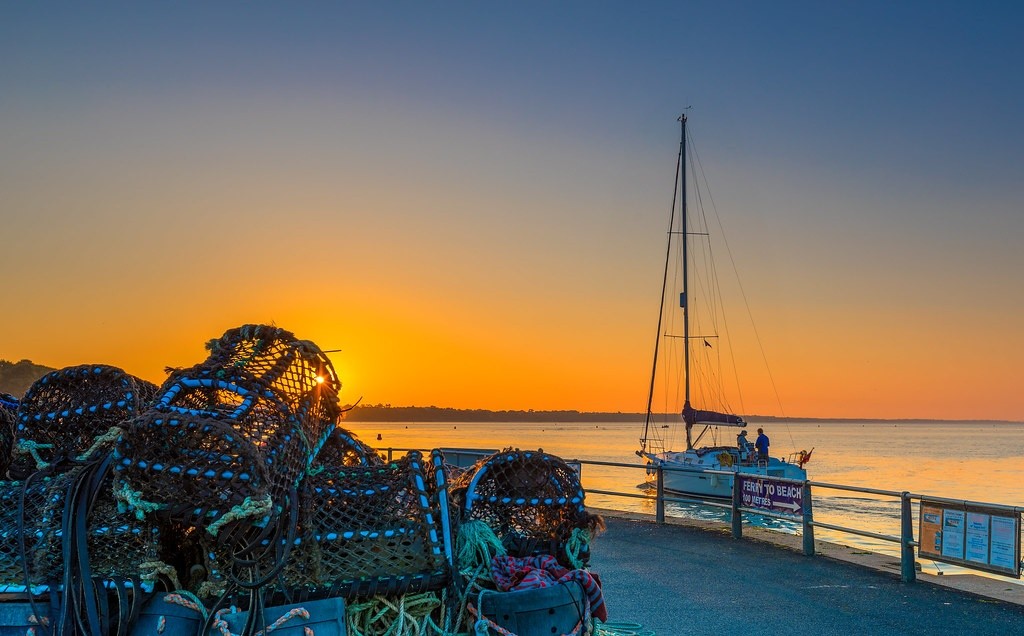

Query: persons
[737,430,749,462]
[755,428,770,465]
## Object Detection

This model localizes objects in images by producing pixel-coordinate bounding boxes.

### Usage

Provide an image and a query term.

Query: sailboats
[636,113,806,500]
